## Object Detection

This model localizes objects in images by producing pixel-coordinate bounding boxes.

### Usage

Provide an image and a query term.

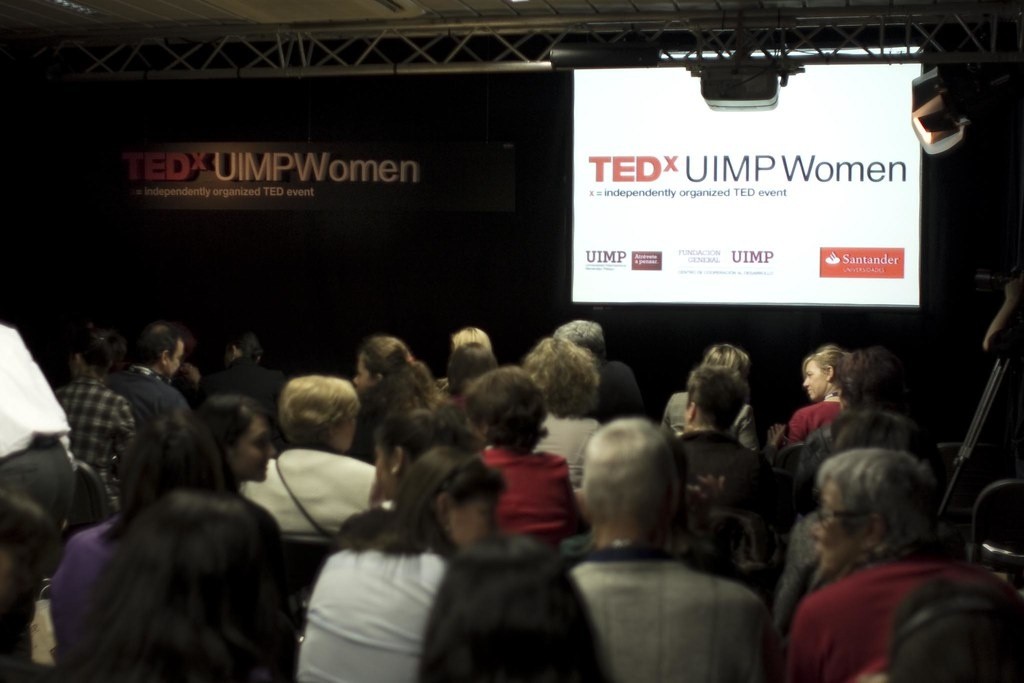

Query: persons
[0,272,1024,683]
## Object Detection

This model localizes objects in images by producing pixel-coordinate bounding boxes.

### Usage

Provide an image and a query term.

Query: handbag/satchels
[29,583,58,669]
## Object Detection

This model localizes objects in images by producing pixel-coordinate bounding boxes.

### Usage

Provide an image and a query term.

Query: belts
[29,438,59,448]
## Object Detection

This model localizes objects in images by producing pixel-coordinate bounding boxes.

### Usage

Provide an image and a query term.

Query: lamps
[911,62,984,154]
[550,41,664,68]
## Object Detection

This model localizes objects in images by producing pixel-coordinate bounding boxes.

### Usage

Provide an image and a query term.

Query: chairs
[283,532,335,587]
[937,442,1024,590]
[75,461,106,531]
[770,442,803,524]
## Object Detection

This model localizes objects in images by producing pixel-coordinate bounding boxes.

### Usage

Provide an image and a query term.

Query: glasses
[817,506,869,532]
[225,394,257,446]
